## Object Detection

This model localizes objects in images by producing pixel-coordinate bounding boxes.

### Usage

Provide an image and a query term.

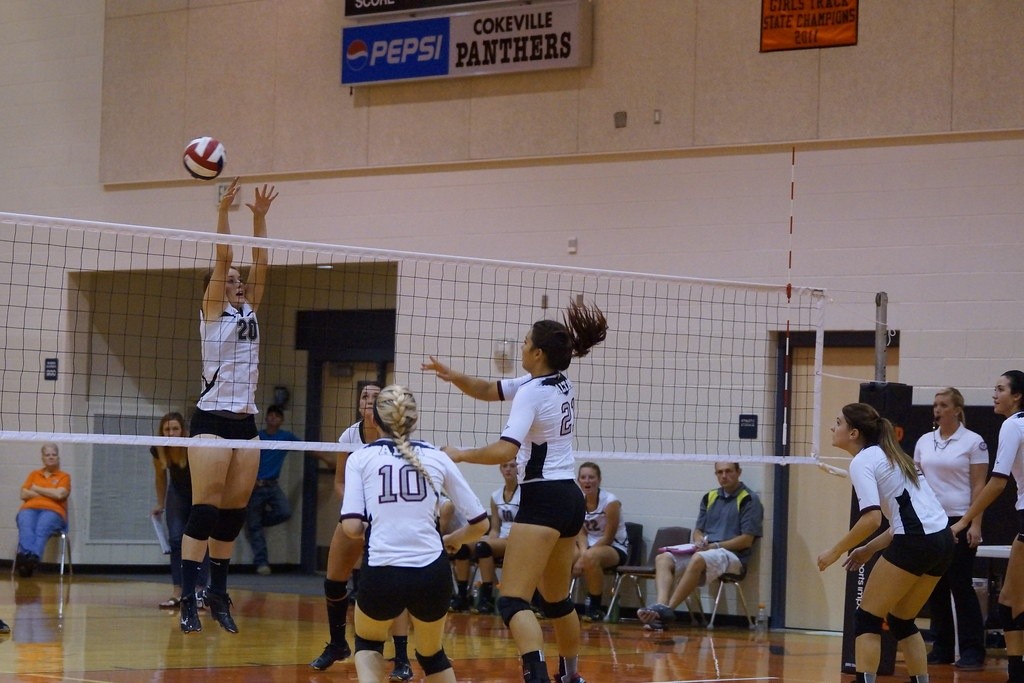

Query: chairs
[460,562,505,610]
[691,532,757,632]
[12,527,72,578]
[601,527,692,621]
[570,522,643,612]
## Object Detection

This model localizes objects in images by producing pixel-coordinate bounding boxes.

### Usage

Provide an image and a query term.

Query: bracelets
[715,542,720,548]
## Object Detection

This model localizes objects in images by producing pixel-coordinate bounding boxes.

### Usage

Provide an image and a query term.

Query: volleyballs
[183,137,227,181]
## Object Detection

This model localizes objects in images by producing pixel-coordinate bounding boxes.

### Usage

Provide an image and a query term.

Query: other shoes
[579,610,603,622]
[15,551,38,579]
[0,619,10,633]
[534,611,545,619]
[257,564,272,576]
[552,672,586,683]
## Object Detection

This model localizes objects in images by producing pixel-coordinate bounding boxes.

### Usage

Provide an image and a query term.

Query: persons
[150,413,208,587]
[245,405,337,576]
[420,301,608,683]
[819,403,955,683]
[180,176,279,634]
[572,463,628,621]
[15,444,71,577]
[310,382,414,683]
[913,388,988,668]
[449,459,525,612]
[436,493,466,535]
[636,462,763,630]
[339,385,489,683]
[950,369,1024,683]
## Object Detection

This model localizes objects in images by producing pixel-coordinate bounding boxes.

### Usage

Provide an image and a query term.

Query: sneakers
[197,585,238,634]
[927,651,954,665]
[179,596,202,633]
[955,653,983,667]
[310,640,351,670]
[644,622,669,632]
[448,596,473,613]
[470,598,494,614]
[637,602,675,623]
[388,659,414,681]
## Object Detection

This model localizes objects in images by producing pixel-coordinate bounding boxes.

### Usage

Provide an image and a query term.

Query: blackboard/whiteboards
[83,413,195,545]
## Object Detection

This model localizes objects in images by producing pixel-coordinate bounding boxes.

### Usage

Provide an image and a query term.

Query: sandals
[159,596,183,609]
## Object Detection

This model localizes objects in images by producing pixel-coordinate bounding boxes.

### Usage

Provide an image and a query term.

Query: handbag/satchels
[986,631,1007,648]
